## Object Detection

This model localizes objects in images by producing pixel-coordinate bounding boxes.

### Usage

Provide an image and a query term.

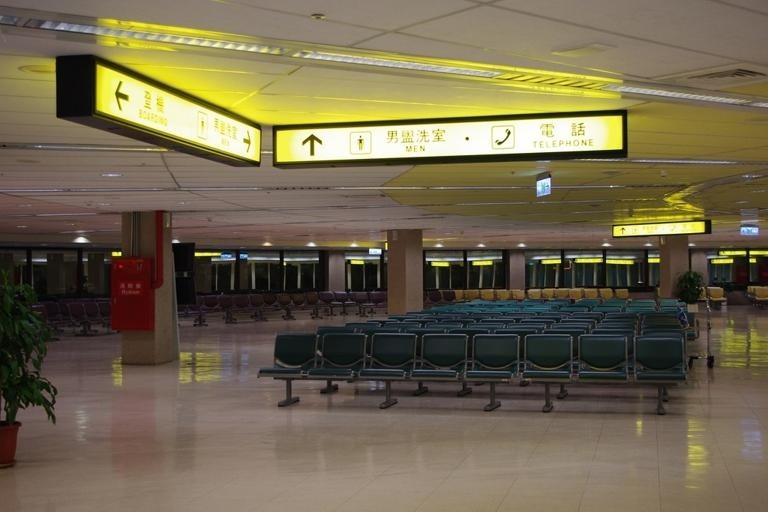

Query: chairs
[31,297,116,342]
[747,288,766,306]
[191,289,387,327]
[693,288,727,310]
[436,288,631,303]
[257,299,693,417]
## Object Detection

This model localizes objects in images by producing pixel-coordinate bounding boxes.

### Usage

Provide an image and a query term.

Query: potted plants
[0,263,63,468]
